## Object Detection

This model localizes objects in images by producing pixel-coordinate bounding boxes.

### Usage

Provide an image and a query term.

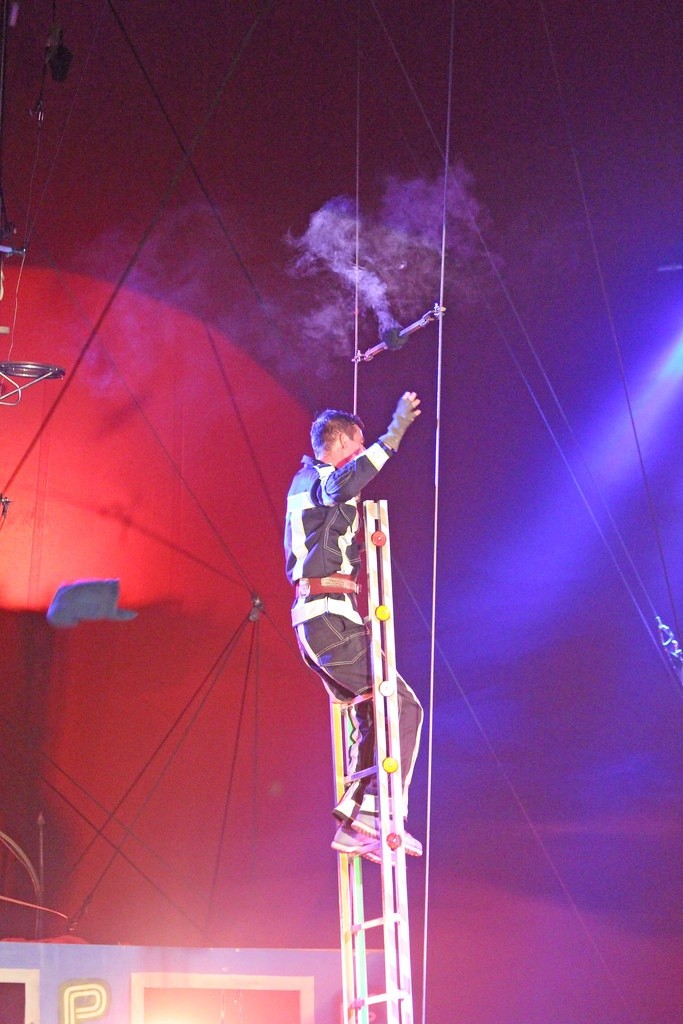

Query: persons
[285,389,428,866]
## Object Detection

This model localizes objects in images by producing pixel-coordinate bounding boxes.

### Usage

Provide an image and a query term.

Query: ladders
[328,500,415,1024]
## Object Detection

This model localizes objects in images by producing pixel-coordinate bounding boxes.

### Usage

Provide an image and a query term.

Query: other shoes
[350,812,423,856]
[331,821,396,866]
